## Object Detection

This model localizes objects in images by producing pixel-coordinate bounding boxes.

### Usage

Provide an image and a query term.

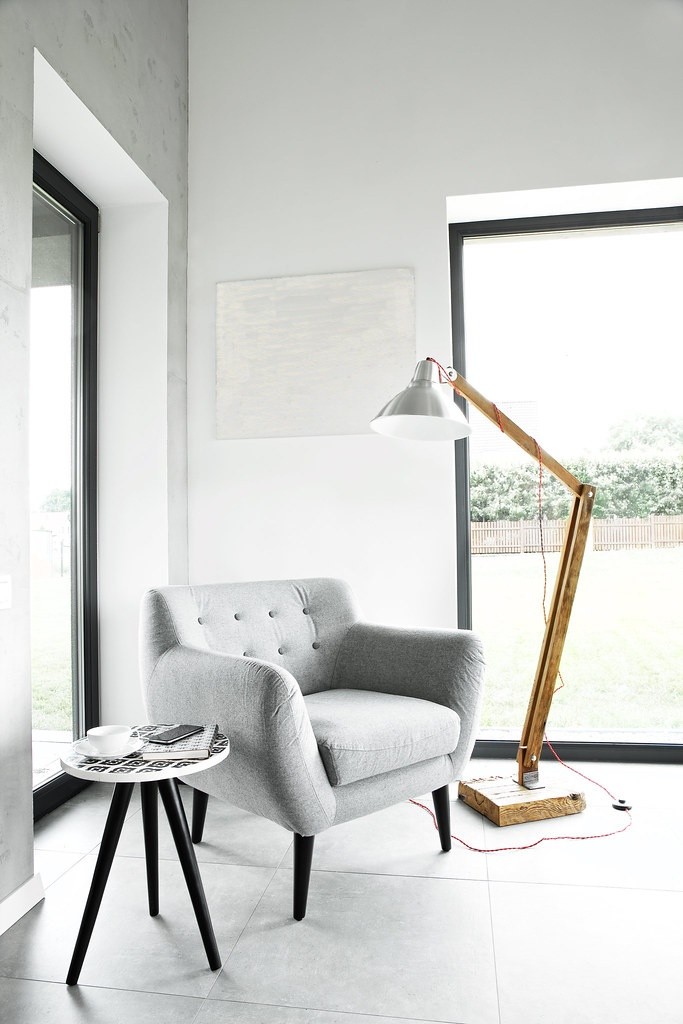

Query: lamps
[370,355,594,826]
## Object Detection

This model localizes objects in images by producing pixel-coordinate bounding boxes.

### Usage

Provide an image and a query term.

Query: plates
[75,738,145,760]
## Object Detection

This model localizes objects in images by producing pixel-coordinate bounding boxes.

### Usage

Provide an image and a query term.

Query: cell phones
[149,725,204,745]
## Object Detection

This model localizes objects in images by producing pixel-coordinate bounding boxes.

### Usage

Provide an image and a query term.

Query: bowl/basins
[87,725,130,753]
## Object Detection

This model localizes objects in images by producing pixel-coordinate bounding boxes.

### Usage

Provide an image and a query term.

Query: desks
[57,723,230,975]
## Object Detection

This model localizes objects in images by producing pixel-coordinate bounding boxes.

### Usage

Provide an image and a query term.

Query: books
[142,722,219,761]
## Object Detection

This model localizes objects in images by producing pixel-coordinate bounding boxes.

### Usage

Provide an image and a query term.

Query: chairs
[146,579,483,916]
